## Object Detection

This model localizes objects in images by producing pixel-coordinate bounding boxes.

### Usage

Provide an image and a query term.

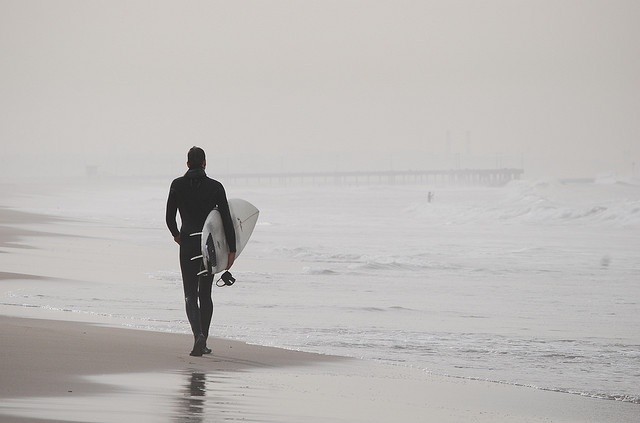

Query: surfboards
[190,197,260,275]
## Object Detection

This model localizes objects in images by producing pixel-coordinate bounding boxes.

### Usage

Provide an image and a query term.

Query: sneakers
[190,334,206,356]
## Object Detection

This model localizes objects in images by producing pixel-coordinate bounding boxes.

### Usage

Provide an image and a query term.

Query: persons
[165,147,236,357]
